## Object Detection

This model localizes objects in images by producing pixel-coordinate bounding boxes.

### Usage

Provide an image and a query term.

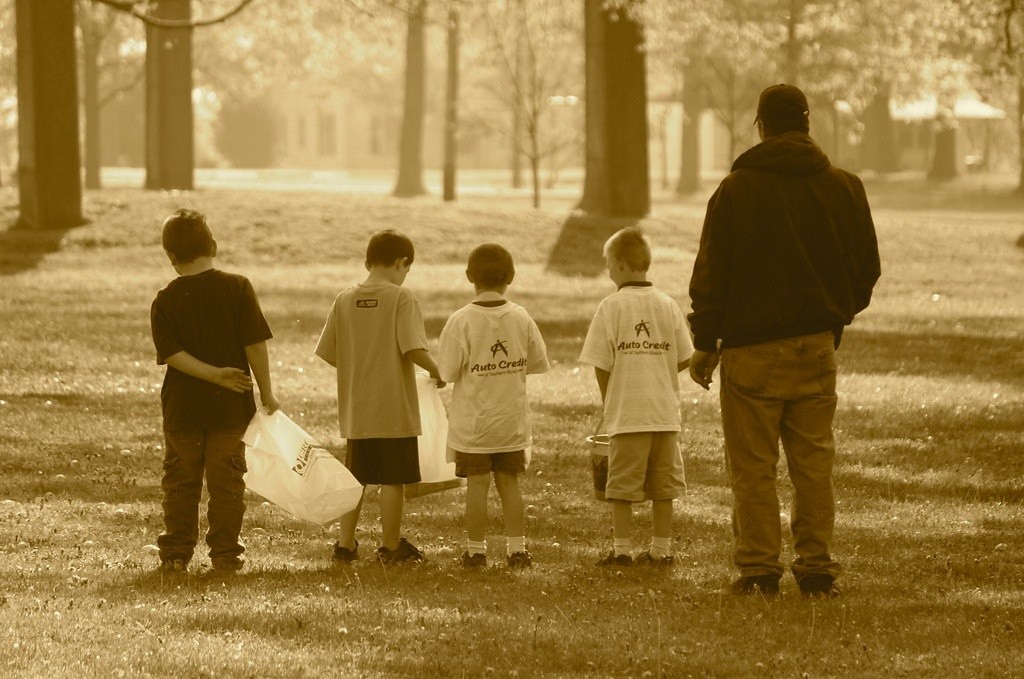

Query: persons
[436,242,552,570]
[578,226,693,570]
[689,85,881,597]
[150,208,281,571]
[313,229,445,565]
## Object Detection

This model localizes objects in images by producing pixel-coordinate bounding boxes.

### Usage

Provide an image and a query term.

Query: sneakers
[377,537,439,567]
[506,551,537,572]
[449,550,487,570]
[594,549,632,567]
[331,538,358,563]
[648,550,674,568]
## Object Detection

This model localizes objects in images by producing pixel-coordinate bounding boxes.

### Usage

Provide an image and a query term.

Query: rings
[704,377,707,380]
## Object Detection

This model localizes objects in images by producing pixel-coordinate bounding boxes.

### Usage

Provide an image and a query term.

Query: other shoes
[211,552,246,570]
[161,556,190,573]
[799,574,842,600]
[727,575,779,595]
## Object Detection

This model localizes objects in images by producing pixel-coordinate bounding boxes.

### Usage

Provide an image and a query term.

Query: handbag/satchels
[404,371,462,499]
[242,406,364,526]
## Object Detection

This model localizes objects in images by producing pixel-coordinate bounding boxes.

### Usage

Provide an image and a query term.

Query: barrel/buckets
[585,418,610,502]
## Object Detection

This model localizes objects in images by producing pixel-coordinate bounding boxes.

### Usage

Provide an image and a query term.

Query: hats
[753,84,809,125]
